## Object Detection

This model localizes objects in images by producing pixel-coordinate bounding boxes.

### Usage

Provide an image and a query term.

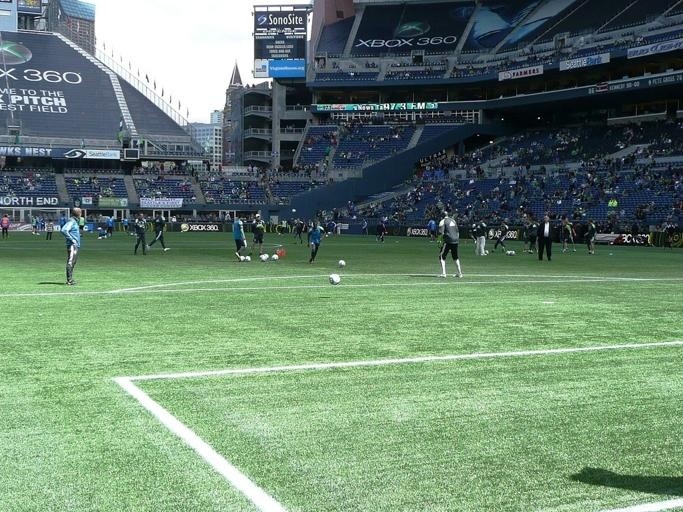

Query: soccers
[339,260,345,266]
[240,253,280,262]
[329,273,340,284]
[506,250,515,256]
[485,250,489,255]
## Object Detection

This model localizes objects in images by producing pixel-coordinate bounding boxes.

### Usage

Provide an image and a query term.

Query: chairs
[0,14,683,231]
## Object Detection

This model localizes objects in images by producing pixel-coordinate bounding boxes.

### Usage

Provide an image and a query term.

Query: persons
[307,115,405,165]
[269,200,340,264]
[1,206,66,240]
[133,211,171,256]
[60,208,82,284]
[229,213,266,262]
[171,213,233,222]
[333,35,645,80]
[346,115,683,261]
[439,210,463,279]
[79,214,130,240]
[1,162,334,203]
